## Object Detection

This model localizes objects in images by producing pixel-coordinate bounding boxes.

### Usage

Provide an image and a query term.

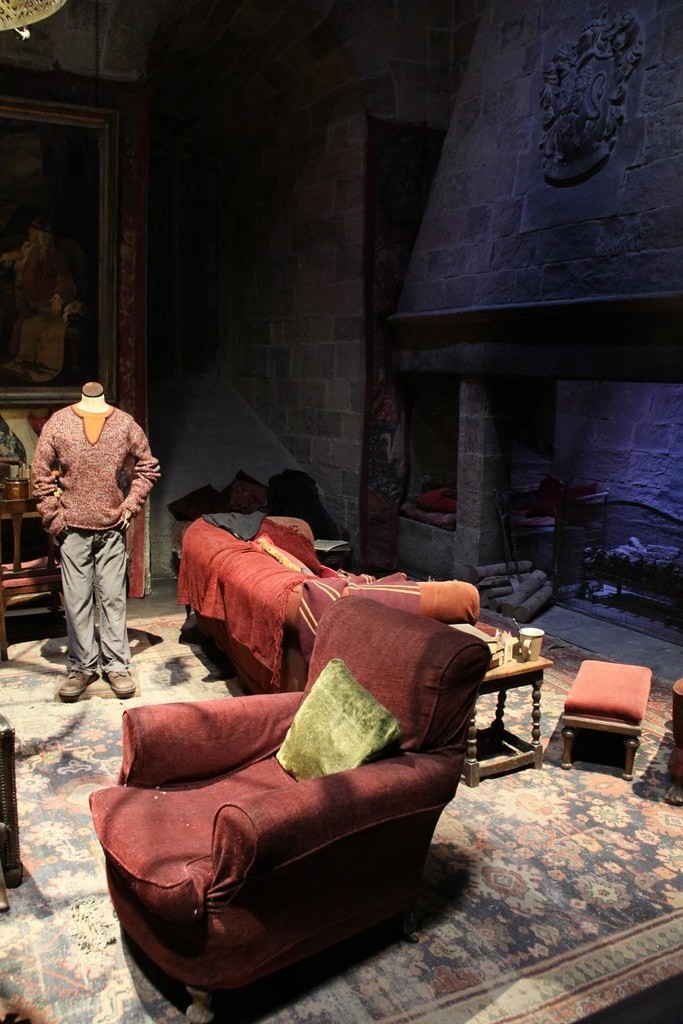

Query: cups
[518,627,544,662]
[5,474,29,500]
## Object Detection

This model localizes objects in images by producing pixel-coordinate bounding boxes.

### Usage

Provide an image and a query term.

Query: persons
[30,382,160,696]
[0,202,75,360]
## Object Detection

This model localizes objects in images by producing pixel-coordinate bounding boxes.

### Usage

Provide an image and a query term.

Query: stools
[560,659,652,780]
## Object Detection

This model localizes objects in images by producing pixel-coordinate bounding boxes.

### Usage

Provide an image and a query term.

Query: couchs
[88,596,493,1024]
[177,511,503,696]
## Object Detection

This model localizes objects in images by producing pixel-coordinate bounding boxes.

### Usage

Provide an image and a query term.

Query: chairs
[0,499,68,661]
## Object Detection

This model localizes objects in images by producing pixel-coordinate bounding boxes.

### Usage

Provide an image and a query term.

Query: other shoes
[107,671,136,694]
[59,672,93,696]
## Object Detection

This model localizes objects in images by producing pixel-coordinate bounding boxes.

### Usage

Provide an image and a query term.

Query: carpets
[0,610,683,1024]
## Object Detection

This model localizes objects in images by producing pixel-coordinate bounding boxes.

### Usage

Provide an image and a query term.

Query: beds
[396,498,604,588]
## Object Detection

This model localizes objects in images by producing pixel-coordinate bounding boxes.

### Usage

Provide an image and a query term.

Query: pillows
[415,486,459,513]
[523,476,598,526]
[275,658,400,783]
[258,538,315,576]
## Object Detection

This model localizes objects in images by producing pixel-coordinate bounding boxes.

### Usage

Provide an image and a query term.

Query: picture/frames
[0,94,120,405]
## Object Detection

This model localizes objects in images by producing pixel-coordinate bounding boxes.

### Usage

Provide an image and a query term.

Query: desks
[461,654,554,788]
